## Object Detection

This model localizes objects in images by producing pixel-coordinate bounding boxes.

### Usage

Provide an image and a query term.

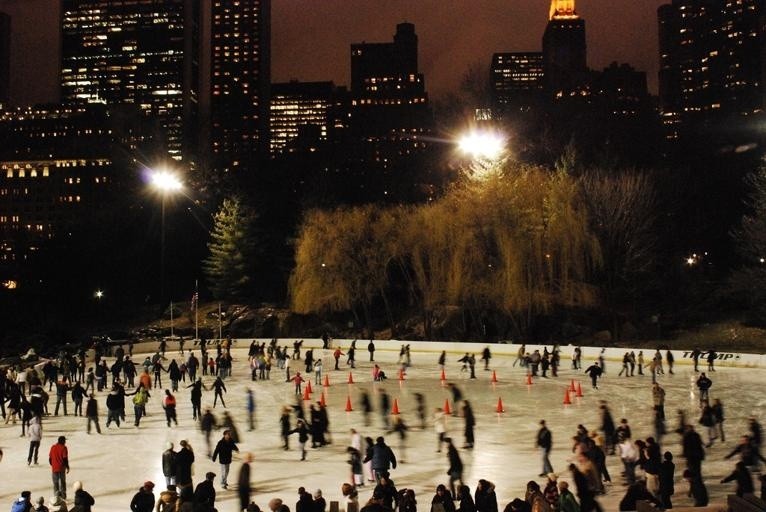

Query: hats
[35,496,44,503]
[267,499,282,511]
[547,472,560,482]
[48,496,67,512]
[558,482,568,490]
[167,441,174,450]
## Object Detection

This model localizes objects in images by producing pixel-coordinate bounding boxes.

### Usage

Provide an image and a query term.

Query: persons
[1,332,765,511]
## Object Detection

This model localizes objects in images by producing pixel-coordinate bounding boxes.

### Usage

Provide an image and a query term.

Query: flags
[189,284,197,311]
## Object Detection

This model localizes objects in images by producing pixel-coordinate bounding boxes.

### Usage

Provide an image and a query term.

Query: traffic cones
[324,375,331,387]
[490,370,498,382]
[440,369,448,380]
[319,392,327,408]
[399,369,405,380]
[303,379,312,400]
[347,372,354,384]
[444,399,452,414]
[391,398,401,415]
[563,378,583,405]
[496,396,505,413]
[344,396,353,411]
[525,371,535,384]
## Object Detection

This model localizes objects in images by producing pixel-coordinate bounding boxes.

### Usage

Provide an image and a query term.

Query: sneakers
[221,483,228,488]
